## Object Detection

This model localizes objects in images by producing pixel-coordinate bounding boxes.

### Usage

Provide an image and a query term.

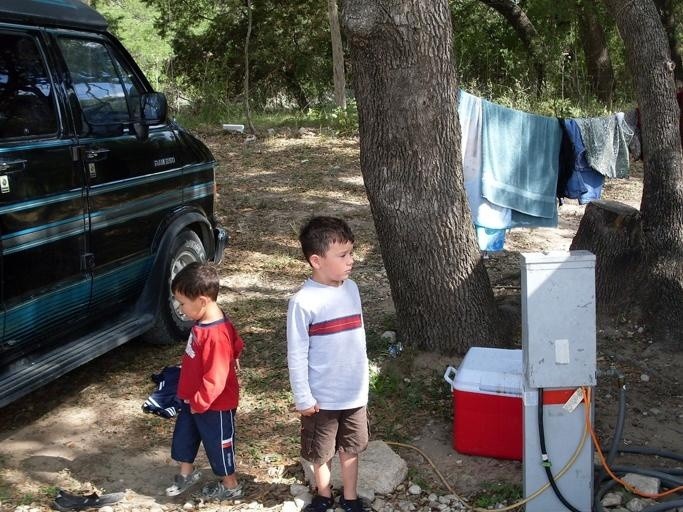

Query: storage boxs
[443,347,523,461]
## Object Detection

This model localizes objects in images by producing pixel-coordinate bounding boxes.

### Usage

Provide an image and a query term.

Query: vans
[0,0,228,405]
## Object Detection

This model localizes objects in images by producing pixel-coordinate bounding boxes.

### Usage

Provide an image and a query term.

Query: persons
[283,215,370,512]
[160,261,244,501]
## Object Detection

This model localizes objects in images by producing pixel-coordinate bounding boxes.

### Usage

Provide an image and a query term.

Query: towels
[459,89,512,250]
[483,100,564,228]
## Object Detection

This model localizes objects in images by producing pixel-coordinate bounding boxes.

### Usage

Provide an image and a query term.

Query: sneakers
[201,480,246,501]
[166,466,202,496]
[53,489,125,511]
[339,494,364,511]
[300,492,333,512]
